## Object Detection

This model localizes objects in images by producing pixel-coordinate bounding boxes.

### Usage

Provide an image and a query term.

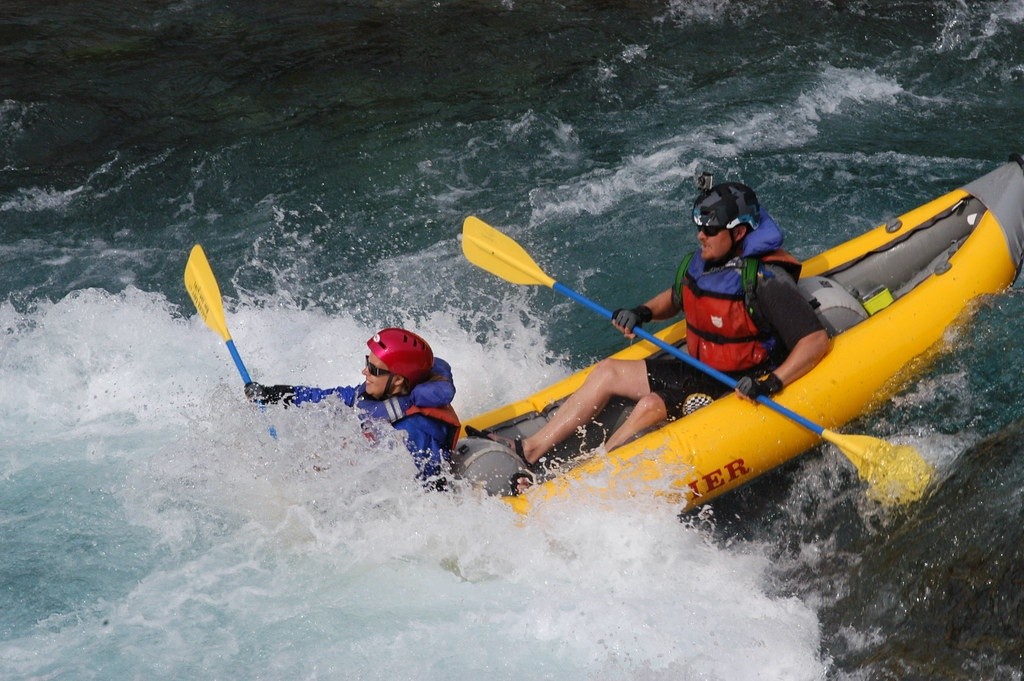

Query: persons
[464,181,833,497]
[245,326,461,500]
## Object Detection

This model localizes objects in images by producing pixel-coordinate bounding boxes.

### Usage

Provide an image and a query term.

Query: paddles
[461,216,930,503]
[184,244,276,438]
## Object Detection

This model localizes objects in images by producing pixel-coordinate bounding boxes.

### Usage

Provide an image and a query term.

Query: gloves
[243,382,294,410]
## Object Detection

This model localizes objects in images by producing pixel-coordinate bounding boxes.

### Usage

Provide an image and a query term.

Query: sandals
[465,425,533,496]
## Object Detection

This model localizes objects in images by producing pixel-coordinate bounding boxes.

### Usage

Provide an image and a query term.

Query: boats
[459,153,1023,511]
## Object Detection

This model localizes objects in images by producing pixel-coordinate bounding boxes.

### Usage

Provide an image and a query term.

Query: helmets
[691,183,761,232]
[366,327,434,393]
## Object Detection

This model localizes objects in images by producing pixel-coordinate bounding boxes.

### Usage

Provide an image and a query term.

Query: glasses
[696,224,727,236]
[364,354,391,378]
